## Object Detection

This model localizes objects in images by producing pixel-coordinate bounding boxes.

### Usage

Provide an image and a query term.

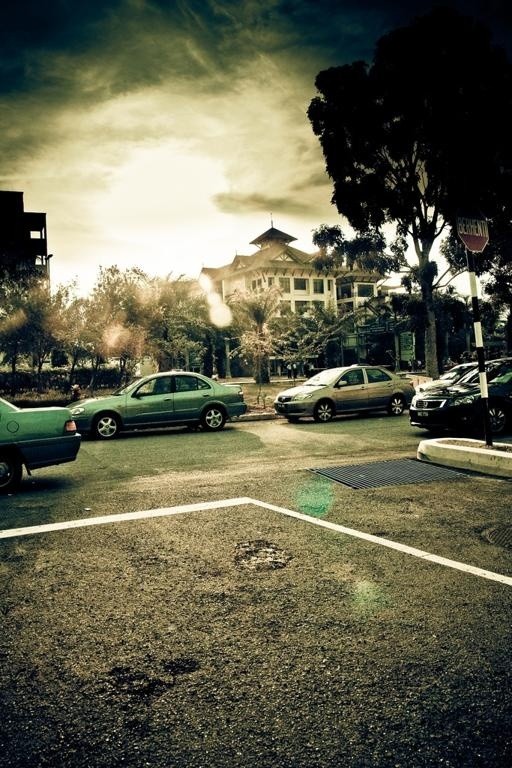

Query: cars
[270,351,512,440]
[0,394,86,492]
[66,366,251,440]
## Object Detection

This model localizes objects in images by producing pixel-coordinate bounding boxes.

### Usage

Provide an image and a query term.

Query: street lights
[43,251,54,304]
